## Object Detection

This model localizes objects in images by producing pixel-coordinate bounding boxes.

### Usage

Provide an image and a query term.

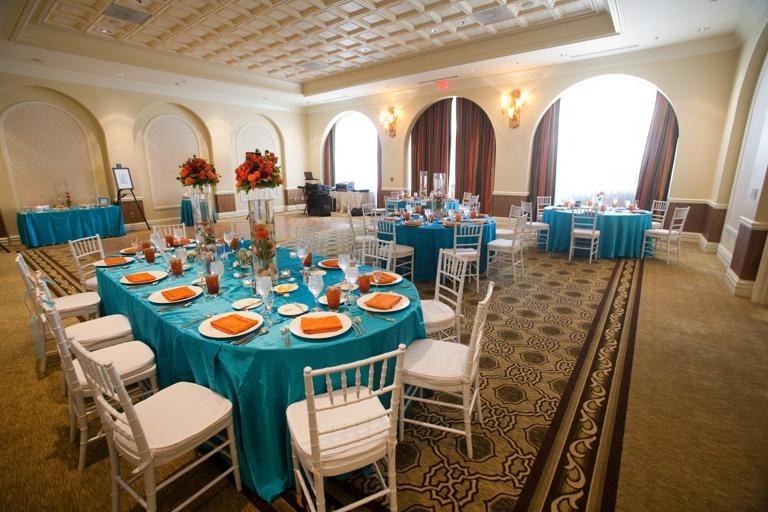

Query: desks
[329,190,376,215]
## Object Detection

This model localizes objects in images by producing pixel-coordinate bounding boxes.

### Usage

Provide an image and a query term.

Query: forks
[365,312,397,322]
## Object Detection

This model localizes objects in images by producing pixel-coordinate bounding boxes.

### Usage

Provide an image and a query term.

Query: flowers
[176,153,220,252]
[234,149,284,284]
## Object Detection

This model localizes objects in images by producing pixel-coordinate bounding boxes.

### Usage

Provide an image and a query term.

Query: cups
[554,198,640,215]
[390,190,455,202]
[358,276,371,296]
[326,285,341,314]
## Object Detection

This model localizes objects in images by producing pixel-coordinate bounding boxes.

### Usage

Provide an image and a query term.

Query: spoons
[280,326,290,345]
[240,326,269,346]
[353,315,366,335]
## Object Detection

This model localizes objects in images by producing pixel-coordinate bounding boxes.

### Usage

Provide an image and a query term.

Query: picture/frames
[111,166,133,191]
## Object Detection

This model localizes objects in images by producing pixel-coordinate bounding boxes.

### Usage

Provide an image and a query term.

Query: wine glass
[130,227,313,315]
[344,265,358,301]
[309,272,324,312]
[372,260,381,292]
[338,254,351,287]
[385,202,489,227]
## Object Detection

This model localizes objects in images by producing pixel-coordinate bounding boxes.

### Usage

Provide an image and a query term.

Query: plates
[360,270,402,286]
[92,256,134,268]
[290,311,352,339]
[318,294,347,306]
[197,310,264,339]
[317,257,357,270]
[119,270,168,286]
[311,268,326,276]
[120,247,154,254]
[358,291,409,313]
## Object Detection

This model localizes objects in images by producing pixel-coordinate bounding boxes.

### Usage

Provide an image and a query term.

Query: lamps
[500,89,527,128]
[377,107,397,138]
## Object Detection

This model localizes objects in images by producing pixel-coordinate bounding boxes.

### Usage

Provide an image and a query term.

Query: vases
[247,189,278,285]
[190,184,216,254]
